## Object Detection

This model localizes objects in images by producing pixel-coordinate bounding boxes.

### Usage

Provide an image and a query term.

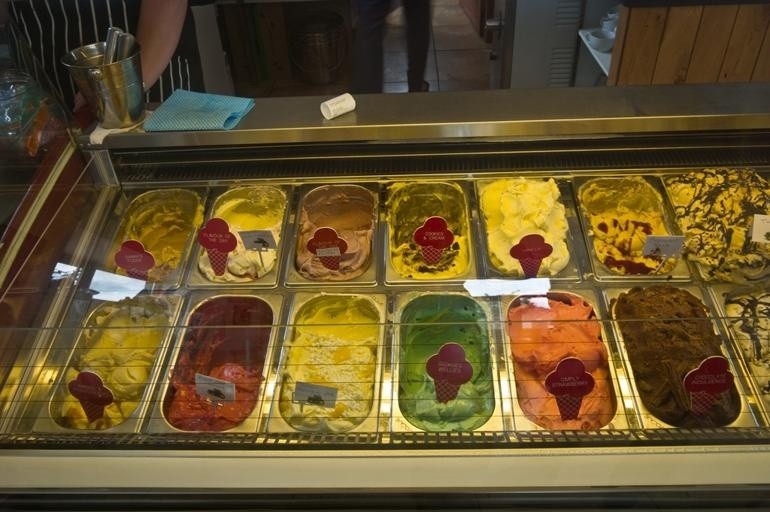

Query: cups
[586,10,618,52]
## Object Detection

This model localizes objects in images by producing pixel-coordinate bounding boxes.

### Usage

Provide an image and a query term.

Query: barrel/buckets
[59,41,145,130]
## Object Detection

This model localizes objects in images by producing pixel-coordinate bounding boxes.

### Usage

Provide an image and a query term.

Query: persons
[1,0,204,116]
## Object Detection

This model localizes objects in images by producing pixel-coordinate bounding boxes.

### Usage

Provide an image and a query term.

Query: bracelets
[140,79,153,95]
